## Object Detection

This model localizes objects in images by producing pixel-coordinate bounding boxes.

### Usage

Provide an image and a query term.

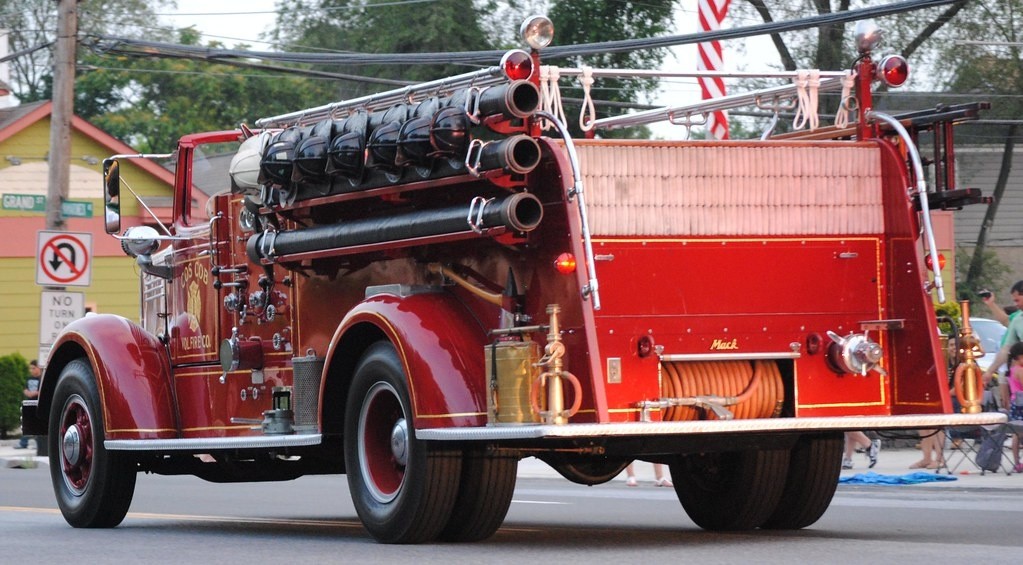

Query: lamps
[81,157,99,165]
[9,157,22,166]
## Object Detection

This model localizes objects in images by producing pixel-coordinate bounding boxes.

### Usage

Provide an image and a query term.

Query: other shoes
[13,444,24,449]
[909,461,931,469]
[927,462,946,469]
[1014,464,1023,472]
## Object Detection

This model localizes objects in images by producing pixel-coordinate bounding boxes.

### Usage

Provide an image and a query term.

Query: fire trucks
[16,16,1008,545]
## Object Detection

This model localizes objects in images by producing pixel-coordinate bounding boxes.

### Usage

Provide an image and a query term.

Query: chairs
[933,387,1023,476]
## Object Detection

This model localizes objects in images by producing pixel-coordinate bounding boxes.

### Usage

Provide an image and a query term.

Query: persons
[105,160,120,223]
[945,279,1023,472]
[626,459,672,486]
[843,430,882,469]
[909,430,948,470]
[14,360,44,449]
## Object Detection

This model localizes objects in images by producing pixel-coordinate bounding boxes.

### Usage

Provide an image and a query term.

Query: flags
[697,0,731,140]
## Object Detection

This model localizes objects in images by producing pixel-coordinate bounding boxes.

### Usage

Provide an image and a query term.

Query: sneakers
[842,457,853,469]
[868,439,881,468]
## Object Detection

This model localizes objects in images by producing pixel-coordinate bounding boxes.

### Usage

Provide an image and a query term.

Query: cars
[947,314,1023,440]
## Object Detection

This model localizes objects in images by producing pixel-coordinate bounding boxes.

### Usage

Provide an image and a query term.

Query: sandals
[626,476,637,486]
[655,478,673,487]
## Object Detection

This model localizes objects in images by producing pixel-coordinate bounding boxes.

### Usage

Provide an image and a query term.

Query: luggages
[976,424,1008,475]
